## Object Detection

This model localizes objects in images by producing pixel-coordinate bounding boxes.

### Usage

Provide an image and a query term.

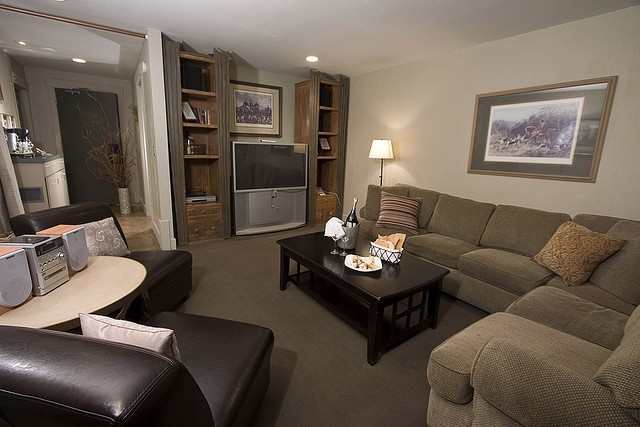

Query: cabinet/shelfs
[162,40,231,245]
[234,190,308,237]
[13,156,68,214]
[295,71,343,228]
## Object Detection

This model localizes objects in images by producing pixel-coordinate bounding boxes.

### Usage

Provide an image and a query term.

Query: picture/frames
[182,100,199,123]
[229,79,283,137]
[466,74,619,183]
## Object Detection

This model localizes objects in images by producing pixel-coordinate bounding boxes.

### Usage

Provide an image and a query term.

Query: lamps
[367,137,395,187]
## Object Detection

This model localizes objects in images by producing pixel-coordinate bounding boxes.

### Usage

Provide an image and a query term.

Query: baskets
[370,245,406,265]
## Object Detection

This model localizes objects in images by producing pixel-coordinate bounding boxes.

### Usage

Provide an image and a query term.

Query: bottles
[346,198,358,228]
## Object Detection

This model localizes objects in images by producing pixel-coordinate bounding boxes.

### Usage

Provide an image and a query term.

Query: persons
[236,98,272,124]
[537,119,546,134]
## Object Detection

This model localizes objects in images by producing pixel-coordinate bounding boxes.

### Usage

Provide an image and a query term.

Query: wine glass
[340,233,350,256]
[330,235,340,255]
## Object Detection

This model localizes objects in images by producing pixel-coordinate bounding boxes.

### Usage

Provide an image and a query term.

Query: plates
[344,254,383,272]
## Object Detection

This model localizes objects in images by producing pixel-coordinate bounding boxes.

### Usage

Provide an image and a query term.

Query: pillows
[81,216,132,257]
[374,190,423,236]
[532,219,628,286]
[77,310,185,363]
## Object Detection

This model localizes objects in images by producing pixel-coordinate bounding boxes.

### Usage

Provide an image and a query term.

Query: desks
[1,253,152,348]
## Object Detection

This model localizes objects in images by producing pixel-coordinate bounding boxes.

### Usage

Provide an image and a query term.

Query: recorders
[0,234,69,295]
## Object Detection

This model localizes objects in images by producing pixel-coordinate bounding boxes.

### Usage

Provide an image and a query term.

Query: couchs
[1,310,276,426]
[358,182,623,313]
[425,220,640,427]
[9,200,193,317]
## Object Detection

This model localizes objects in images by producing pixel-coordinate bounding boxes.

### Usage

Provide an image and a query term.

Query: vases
[117,187,132,215]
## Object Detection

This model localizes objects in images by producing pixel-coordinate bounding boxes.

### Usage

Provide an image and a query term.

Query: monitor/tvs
[231,140,308,193]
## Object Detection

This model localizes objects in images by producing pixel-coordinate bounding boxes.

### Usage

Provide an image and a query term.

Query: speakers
[0,246,32,309]
[36,224,89,273]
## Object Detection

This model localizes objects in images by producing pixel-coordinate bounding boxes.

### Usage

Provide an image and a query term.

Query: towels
[322,216,346,240]
